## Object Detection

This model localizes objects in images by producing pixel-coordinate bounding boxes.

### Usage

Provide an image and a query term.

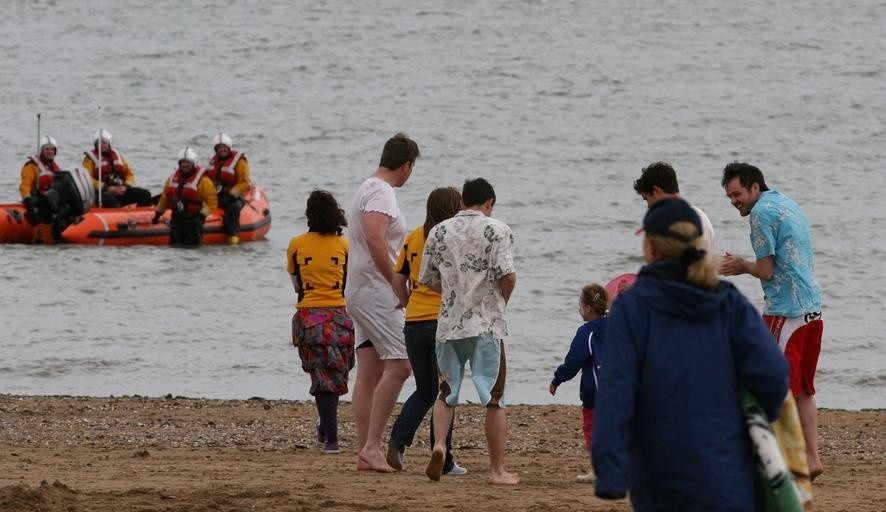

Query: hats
[635,197,703,242]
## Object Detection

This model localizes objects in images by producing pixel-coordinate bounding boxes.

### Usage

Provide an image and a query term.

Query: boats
[1,183,272,248]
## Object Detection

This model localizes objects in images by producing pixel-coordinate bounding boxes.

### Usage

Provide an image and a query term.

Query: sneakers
[321,442,340,453]
[316,421,327,443]
[442,462,468,475]
[577,471,597,484]
[387,439,404,470]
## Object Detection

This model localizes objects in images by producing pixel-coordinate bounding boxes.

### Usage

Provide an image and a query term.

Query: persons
[385,184,466,475]
[416,176,523,486]
[205,132,250,244]
[344,130,421,473]
[286,188,355,454]
[546,283,606,486]
[80,130,151,209]
[18,135,60,199]
[716,160,823,484]
[616,158,714,296]
[151,147,217,247]
[589,196,793,512]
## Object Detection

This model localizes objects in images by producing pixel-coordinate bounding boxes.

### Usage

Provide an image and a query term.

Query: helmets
[177,147,198,170]
[39,136,57,155]
[93,129,112,149]
[213,132,232,153]
